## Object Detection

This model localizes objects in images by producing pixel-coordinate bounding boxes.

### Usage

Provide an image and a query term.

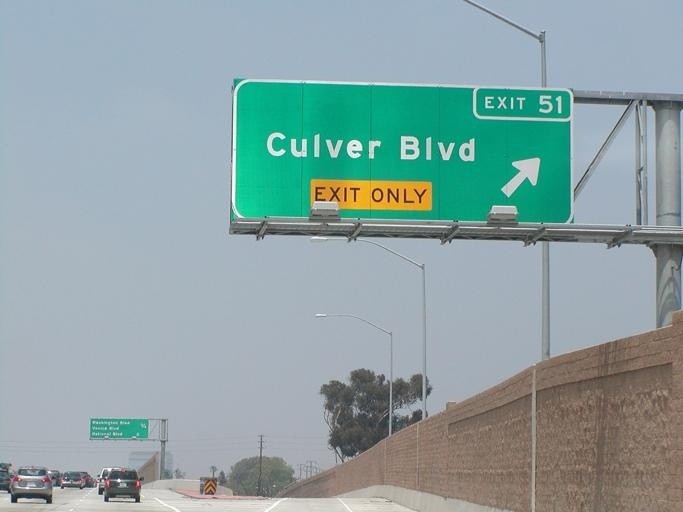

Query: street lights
[465,0,550,359]
[316,314,392,435]
[311,237,426,419]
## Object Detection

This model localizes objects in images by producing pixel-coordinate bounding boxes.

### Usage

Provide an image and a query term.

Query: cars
[103,468,143,502]
[96,467,120,494]
[0,467,96,503]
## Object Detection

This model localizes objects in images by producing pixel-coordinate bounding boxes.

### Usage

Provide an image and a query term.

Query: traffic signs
[230,77,572,226]
